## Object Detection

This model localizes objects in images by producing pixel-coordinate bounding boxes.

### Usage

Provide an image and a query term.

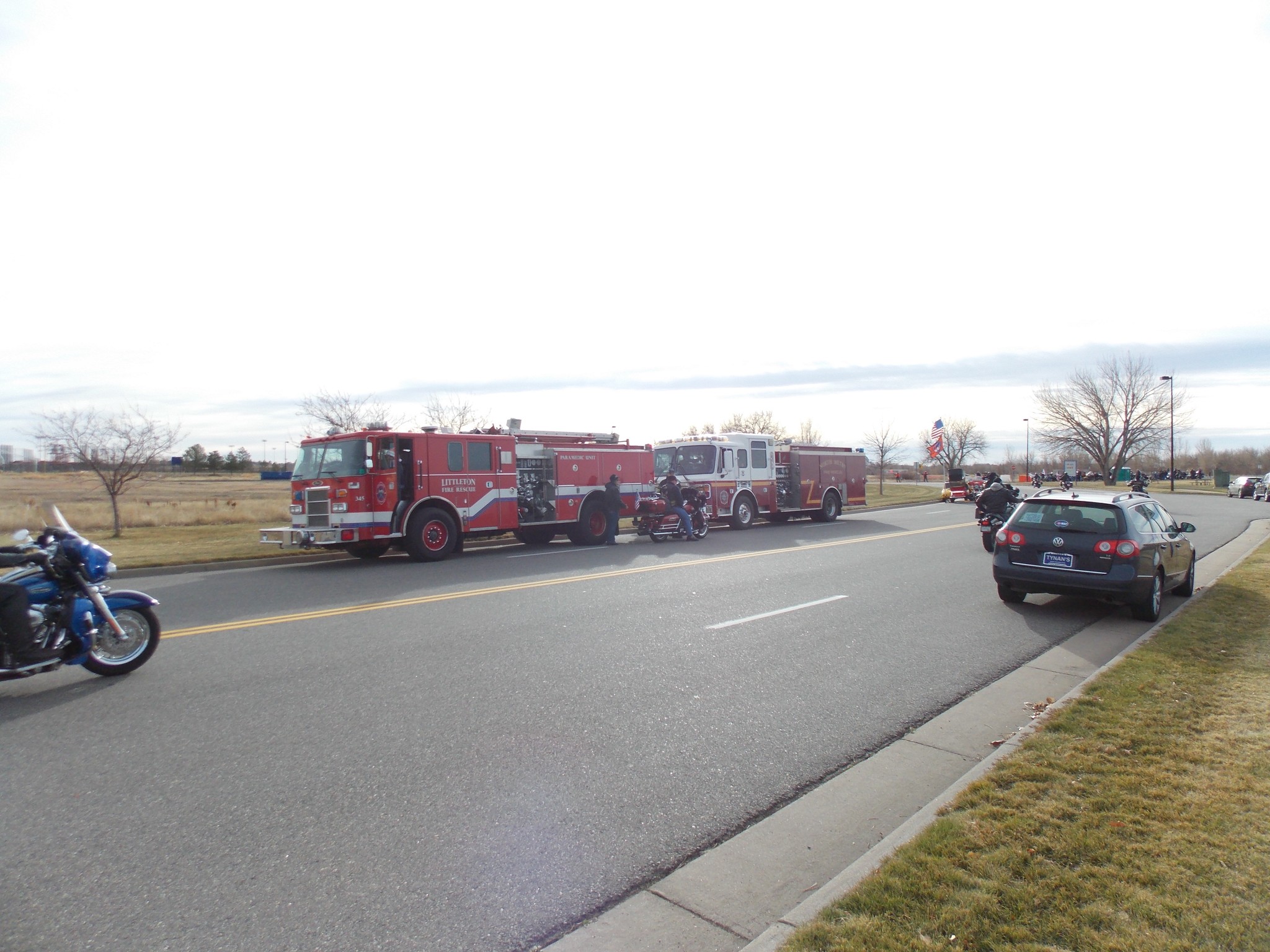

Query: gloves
[28,551,49,566]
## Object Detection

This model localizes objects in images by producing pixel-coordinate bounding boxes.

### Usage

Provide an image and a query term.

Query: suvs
[994,490,1197,623]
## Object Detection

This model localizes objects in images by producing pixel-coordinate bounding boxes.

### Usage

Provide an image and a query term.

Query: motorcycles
[1059,476,1073,490]
[1029,476,1043,488]
[945,468,984,503]
[975,493,1027,553]
[0,504,161,680]
[1039,473,1063,482]
[1076,471,1103,481]
[1129,472,1203,494]
[632,480,712,542]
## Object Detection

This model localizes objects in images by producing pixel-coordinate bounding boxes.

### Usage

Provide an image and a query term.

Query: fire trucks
[257,417,659,564]
[652,433,869,532]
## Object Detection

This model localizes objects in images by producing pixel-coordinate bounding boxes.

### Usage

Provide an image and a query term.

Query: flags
[634,491,641,511]
[927,435,943,458]
[931,419,944,438]
[1040,469,1046,475]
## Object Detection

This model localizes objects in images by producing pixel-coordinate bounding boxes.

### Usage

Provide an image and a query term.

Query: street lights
[1023,419,1029,482]
[1160,376,1174,491]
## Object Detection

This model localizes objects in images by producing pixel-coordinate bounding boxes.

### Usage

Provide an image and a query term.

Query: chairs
[1062,509,1083,527]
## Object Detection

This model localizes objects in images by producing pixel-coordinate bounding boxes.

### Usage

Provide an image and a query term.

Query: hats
[668,469,674,473]
[668,476,676,481]
[610,475,618,481]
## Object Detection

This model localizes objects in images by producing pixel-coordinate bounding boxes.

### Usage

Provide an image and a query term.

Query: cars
[1252,473,1270,502]
[1226,477,1263,499]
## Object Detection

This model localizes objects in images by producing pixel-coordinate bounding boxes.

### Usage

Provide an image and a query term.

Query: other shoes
[687,535,699,541]
[607,541,618,545]
[18,648,62,664]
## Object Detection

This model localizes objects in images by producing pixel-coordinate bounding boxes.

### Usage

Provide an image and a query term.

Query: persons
[894,472,901,482]
[657,469,699,541]
[1157,468,1202,480]
[922,471,929,482]
[1126,470,1150,498]
[972,471,1024,521]
[0,545,57,663]
[1039,472,1070,487]
[1032,473,1040,485]
[1076,469,1081,481]
[602,474,628,545]
[386,451,403,501]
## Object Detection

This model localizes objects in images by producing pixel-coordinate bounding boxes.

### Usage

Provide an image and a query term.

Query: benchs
[1191,482,1202,485]
[1203,482,1213,486]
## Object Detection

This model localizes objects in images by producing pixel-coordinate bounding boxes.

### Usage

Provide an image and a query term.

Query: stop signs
[1011,466,1016,471]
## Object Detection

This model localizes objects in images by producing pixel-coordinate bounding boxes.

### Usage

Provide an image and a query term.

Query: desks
[1191,479,1212,485]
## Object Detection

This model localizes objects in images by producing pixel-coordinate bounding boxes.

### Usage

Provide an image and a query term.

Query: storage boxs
[1070,482,1073,488]
[1062,482,1066,488]
